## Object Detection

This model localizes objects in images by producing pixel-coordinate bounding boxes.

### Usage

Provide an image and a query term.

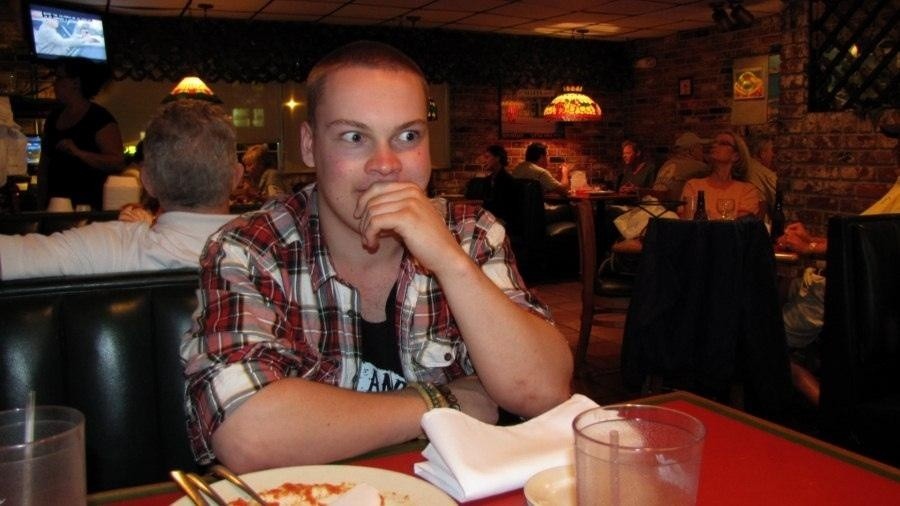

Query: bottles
[690,190,708,220]
[47,197,73,213]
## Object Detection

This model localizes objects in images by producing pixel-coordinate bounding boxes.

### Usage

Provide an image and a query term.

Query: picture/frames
[679,77,694,97]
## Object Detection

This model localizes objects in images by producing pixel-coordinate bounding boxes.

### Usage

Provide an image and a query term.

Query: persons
[2,94,254,281]
[511,142,569,217]
[2,96,29,186]
[178,42,576,474]
[36,13,85,55]
[465,145,536,280]
[618,138,657,188]
[236,144,285,203]
[773,177,900,408]
[678,128,775,221]
[37,60,123,213]
[656,131,712,202]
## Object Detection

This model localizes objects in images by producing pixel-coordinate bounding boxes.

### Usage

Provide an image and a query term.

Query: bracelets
[808,243,818,254]
[405,380,446,413]
[437,380,462,413]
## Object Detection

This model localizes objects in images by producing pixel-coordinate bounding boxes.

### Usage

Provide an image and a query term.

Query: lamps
[542,28,603,124]
[161,2,226,104]
[712,0,753,33]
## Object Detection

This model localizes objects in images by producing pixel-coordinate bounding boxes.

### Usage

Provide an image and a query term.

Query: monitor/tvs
[23,132,42,166]
[29,6,111,63]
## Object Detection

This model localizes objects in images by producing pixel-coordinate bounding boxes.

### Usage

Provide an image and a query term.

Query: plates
[168,463,458,506]
[525,465,576,506]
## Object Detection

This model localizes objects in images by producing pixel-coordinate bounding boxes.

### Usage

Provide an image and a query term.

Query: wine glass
[715,196,736,220]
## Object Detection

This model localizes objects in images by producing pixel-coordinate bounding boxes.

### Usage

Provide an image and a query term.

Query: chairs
[1,175,900,505]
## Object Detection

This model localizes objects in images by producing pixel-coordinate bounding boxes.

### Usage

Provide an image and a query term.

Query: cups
[683,194,697,219]
[2,407,86,506]
[572,402,704,506]
